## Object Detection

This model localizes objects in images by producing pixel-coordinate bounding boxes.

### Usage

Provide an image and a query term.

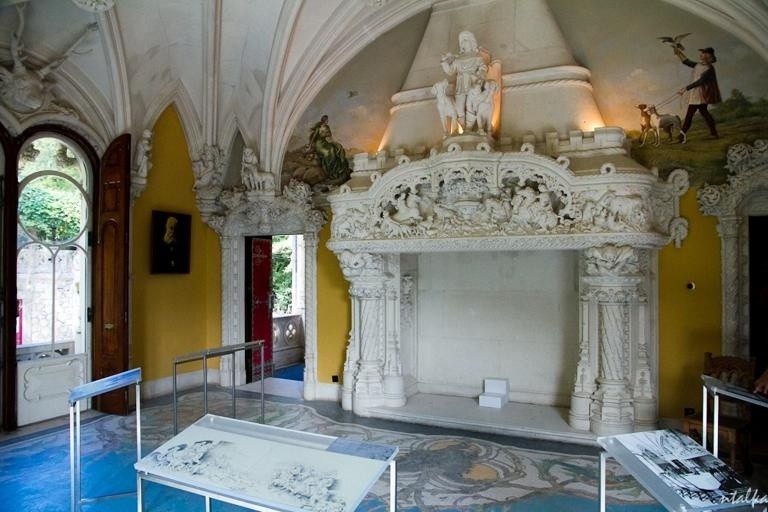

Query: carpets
[0,391,664,512]
[274,363,304,380]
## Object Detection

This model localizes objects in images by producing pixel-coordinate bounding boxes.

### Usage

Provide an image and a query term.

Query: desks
[598,430,767,511]
[132,413,399,512]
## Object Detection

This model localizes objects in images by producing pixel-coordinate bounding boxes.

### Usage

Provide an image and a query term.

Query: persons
[239,144,262,190]
[306,114,346,178]
[431,29,497,134]
[134,129,153,173]
[670,45,721,144]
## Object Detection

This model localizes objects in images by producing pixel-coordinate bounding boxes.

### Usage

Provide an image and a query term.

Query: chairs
[678,351,757,470]
